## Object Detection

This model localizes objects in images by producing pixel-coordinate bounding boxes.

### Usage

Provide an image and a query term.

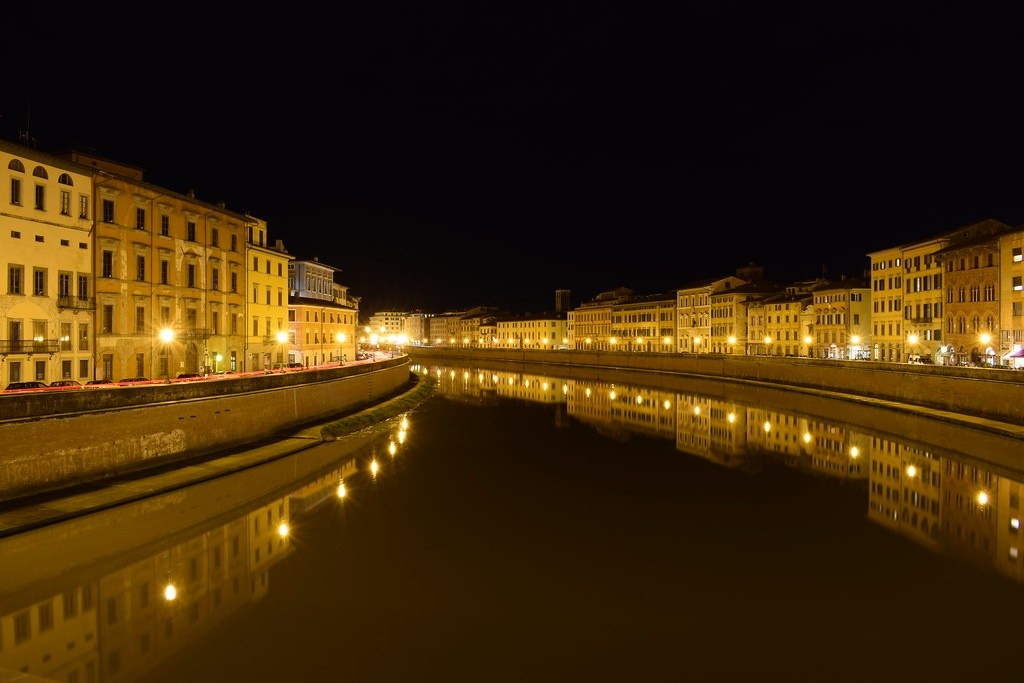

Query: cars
[49,380,82,387]
[357,354,367,360]
[85,379,113,385]
[176,373,201,378]
[118,377,149,382]
[214,370,233,375]
[4,381,49,390]
[263,362,304,372]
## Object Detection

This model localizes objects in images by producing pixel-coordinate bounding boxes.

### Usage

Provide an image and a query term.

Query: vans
[907,354,935,365]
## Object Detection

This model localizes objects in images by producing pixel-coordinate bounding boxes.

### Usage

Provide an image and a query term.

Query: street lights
[163,549,175,601]
[850,336,858,359]
[161,331,174,380]
[280,494,288,536]
[277,333,288,370]
[909,335,917,362]
[981,335,990,365]
[336,334,346,367]
[338,464,344,501]
